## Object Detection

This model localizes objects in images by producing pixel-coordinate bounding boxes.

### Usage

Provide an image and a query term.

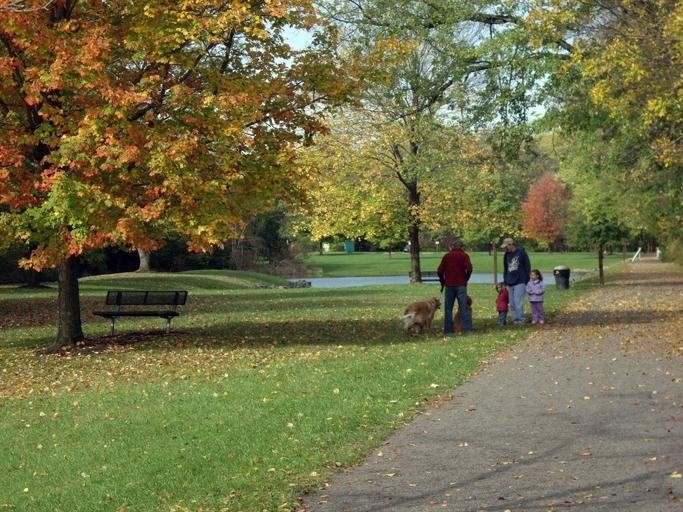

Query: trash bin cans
[345,240,354,253]
[554,265,570,289]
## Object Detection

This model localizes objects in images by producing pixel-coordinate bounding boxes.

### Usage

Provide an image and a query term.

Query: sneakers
[496,319,544,326]
[445,330,474,337]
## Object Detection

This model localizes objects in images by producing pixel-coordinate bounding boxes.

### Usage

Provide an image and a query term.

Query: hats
[500,238,513,249]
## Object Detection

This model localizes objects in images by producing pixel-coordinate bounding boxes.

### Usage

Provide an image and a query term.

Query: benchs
[409,271,441,283]
[91,290,187,346]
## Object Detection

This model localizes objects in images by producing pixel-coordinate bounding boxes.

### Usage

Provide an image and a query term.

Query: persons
[500,238,531,326]
[525,269,545,324]
[437,241,472,334]
[496,283,509,326]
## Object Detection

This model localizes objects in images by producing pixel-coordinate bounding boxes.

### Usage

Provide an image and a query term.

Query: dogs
[452,295,473,334]
[399,297,442,337]
